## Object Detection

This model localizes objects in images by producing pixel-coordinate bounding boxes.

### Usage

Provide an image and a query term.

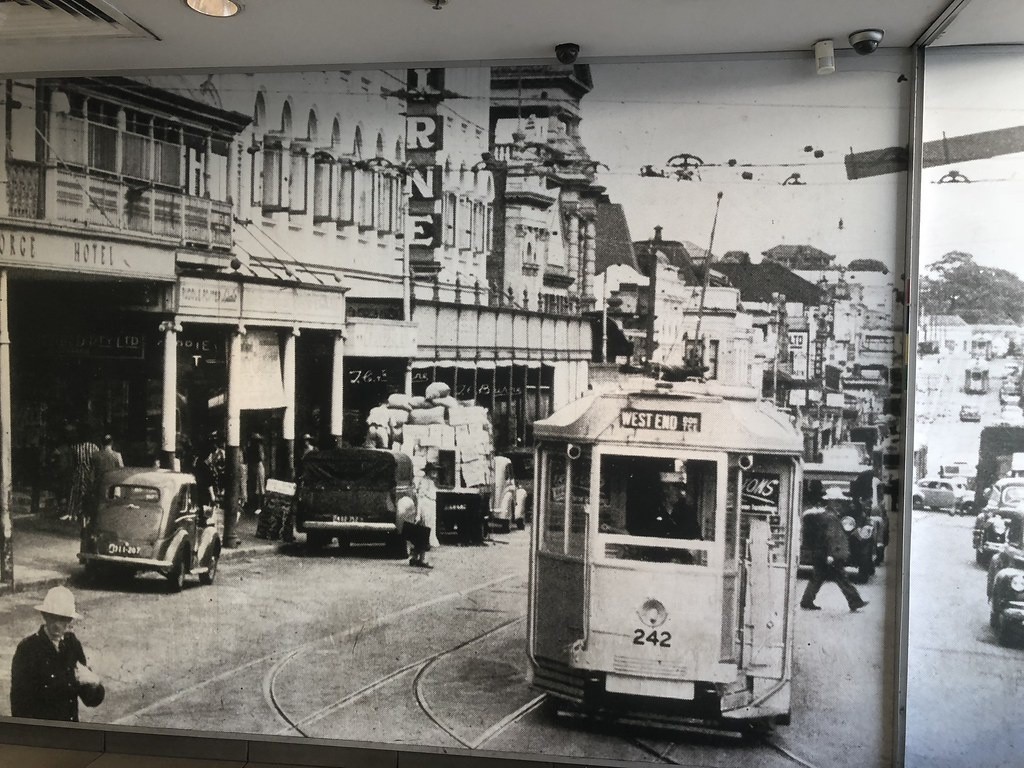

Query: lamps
[812,40,835,77]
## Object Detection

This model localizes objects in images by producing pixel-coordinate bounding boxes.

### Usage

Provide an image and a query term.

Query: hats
[304,433,312,439]
[660,471,686,490]
[822,487,850,501]
[421,462,440,471]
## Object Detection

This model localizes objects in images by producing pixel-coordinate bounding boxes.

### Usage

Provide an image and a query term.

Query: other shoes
[410,558,417,566]
[849,600,868,612]
[800,601,820,610]
[417,560,433,569]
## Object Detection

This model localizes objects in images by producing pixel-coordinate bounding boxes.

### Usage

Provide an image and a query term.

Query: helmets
[34,585,83,623]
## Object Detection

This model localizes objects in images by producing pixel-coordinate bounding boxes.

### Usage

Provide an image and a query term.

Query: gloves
[73,660,100,688]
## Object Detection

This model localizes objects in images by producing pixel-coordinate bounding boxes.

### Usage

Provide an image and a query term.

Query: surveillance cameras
[848,29,886,54]
[554,44,579,64]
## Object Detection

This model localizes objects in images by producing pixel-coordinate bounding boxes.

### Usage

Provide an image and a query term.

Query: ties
[58,640,65,651]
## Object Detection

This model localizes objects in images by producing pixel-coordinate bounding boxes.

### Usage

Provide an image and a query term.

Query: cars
[960,404,981,421]
[294,446,422,559]
[965,367,990,394]
[77,466,221,592]
[998,363,1024,424]
[801,459,890,583]
[912,473,1024,639]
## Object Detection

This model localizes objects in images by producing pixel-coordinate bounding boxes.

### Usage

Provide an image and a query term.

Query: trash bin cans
[256,479,297,542]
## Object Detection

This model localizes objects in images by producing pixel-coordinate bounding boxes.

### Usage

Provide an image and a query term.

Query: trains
[527,378,805,738]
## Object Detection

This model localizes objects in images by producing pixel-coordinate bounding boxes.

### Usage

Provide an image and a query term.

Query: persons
[58,425,319,544]
[631,474,703,564]
[9,591,106,722]
[800,489,869,612]
[408,462,438,568]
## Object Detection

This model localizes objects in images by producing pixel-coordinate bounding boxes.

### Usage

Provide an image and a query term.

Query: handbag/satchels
[401,521,431,549]
